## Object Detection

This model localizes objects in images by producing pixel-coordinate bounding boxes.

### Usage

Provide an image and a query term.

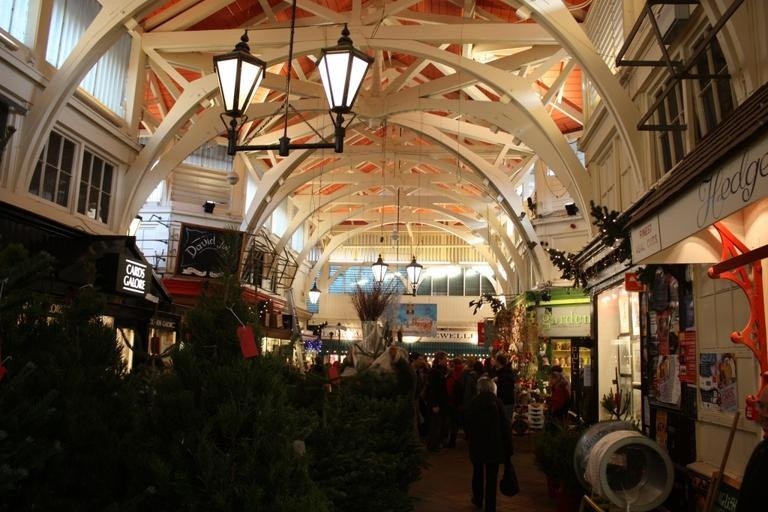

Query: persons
[408,351,426,449]
[495,354,515,430]
[426,352,448,453]
[449,350,494,375]
[464,376,514,511]
[549,365,571,413]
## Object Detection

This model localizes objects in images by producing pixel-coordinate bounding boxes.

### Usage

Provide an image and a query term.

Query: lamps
[315,23,375,154]
[212,27,267,155]
[371,254,388,294]
[202,200,216,212]
[406,254,424,297]
[564,201,579,216]
[308,279,321,305]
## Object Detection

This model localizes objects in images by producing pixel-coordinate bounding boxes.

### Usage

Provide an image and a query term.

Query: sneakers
[471,497,483,508]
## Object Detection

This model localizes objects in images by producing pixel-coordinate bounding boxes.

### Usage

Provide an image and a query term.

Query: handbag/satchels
[500,457,522,497]
